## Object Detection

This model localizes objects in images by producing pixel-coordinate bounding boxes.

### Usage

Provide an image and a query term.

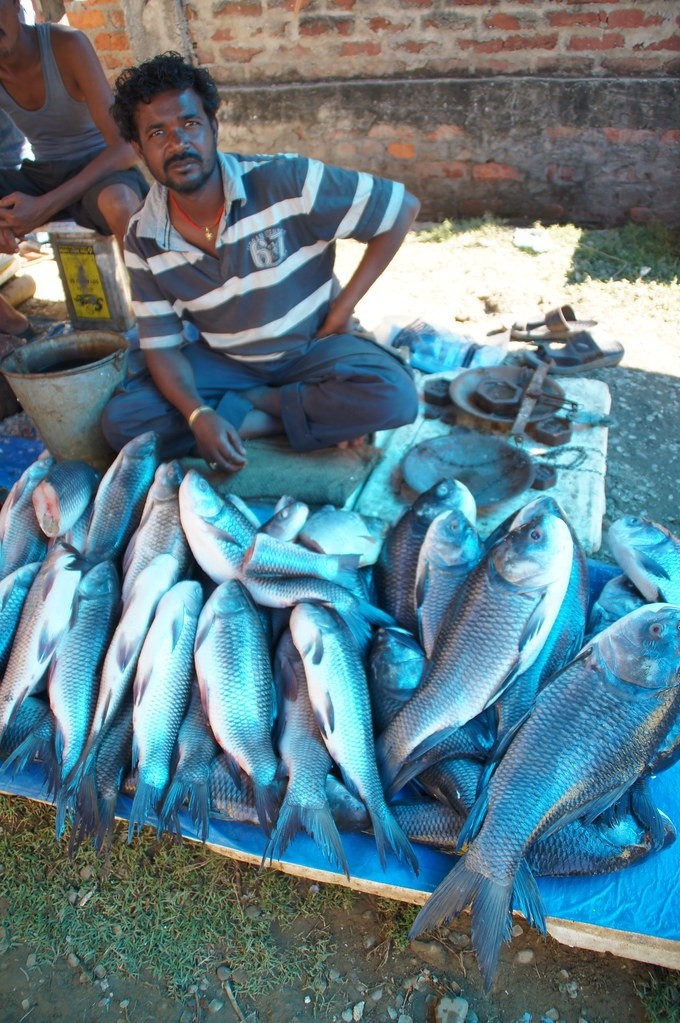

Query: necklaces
[169,193,224,241]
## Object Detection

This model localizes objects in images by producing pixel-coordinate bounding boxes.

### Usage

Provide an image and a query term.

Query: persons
[0,109,26,173]
[0,0,152,265]
[100,50,422,475]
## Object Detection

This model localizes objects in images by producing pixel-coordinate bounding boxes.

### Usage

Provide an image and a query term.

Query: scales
[400,364,577,513]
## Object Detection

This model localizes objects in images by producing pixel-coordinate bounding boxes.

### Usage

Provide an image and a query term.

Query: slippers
[510,304,599,341]
[521,326,625,376]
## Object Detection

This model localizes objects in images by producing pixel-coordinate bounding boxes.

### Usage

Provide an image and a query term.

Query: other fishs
[0,430,680,988]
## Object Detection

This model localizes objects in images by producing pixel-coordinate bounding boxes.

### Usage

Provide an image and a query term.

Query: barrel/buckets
[0,328,130,465]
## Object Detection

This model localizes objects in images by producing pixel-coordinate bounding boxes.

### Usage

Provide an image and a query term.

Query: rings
[209,462,218,470]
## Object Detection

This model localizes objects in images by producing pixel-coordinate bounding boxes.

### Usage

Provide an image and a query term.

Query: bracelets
[188,405,215,430]
